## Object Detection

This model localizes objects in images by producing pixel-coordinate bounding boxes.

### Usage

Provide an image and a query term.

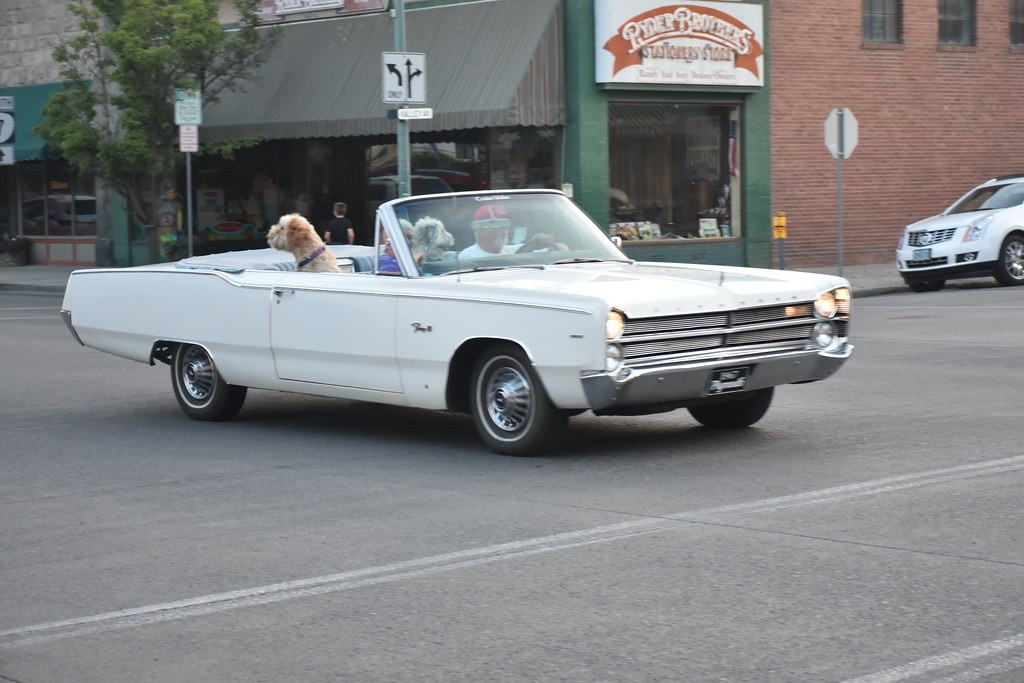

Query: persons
[458,205,570,260]
[325,202,355,246]
[372,218,425,274]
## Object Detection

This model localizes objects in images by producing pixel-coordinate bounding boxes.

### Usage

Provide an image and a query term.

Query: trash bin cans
[5,236,33,267]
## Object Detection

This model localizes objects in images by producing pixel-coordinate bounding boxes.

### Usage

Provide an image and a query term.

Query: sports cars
[56,190,855,458]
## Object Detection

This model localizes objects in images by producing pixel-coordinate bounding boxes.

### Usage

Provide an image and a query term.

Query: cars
[366,173,455,227]
[20,193,96,229]
[894,171,1024,293]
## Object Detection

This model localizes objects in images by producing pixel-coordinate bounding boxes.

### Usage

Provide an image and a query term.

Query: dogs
[411,216,456,261]
[266,213,341,273]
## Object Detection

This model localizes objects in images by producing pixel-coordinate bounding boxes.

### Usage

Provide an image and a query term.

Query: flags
[729,121,738,174]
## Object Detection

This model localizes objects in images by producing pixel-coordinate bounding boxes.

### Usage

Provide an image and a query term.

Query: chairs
[349,255,383,273]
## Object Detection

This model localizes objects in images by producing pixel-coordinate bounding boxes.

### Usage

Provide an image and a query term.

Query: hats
[471,204,513,229]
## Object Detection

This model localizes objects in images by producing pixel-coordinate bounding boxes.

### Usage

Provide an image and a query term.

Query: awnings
[157,0,568,146]
[0,78,93,163]
